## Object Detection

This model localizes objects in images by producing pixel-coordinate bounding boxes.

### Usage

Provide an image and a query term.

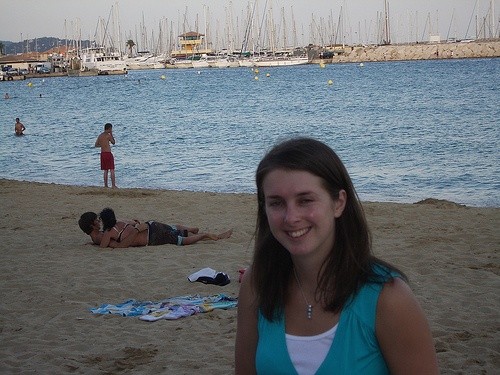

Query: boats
[79,0,310,75]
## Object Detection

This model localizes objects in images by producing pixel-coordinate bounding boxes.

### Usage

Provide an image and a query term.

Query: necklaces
[293,266,318,320]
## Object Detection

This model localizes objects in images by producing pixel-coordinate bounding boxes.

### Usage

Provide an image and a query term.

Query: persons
[5,93,9,98]
[235,137,441,375]
[15,118,25,135]
[79,212,233,244]
[99,207,218,247]
[95,123,118,189]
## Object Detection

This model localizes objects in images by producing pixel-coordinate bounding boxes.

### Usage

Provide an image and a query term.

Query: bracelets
[135,227,140,233]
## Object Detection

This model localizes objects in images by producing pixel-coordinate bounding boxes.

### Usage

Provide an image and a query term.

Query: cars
[34,63,46,73]
[39,67,50,74]
[3,67,30,76]
